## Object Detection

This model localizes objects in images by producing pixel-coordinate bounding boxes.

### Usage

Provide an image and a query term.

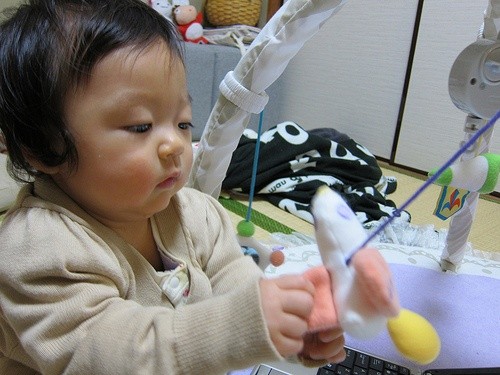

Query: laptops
[218,334,500,374]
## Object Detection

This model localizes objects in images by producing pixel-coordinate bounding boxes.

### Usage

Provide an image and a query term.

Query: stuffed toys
[300,180,442,364]
[148,0,209,44]
[428,154,500,272]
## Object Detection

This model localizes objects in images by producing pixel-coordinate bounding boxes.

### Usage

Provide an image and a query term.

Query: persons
[0,0,346,375]
